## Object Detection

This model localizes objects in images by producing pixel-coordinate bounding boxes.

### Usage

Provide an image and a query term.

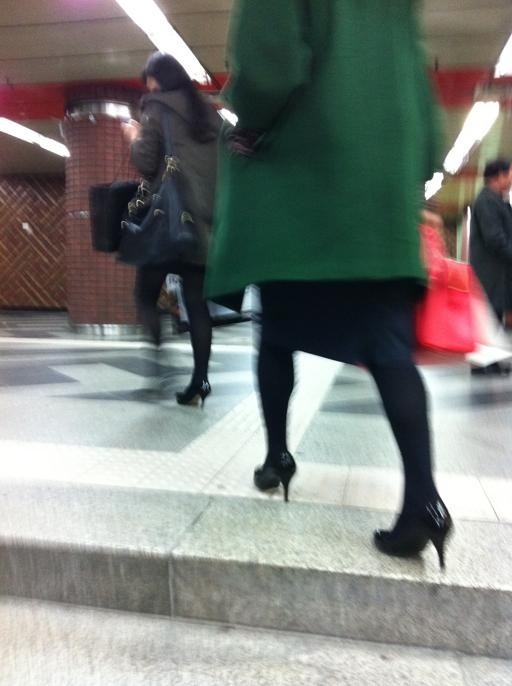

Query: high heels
[253,449,296,504]
[176,375,211,408]
[372,490,452,570]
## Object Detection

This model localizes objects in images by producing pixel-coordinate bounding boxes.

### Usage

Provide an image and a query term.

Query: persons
[199,1,456,575]
[113,48,231,409]
[463,156,512,382]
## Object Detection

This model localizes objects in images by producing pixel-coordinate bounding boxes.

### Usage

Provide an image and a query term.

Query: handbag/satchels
[115,156,200,269]
[414,222,512,367]
[88,176,144,253]
